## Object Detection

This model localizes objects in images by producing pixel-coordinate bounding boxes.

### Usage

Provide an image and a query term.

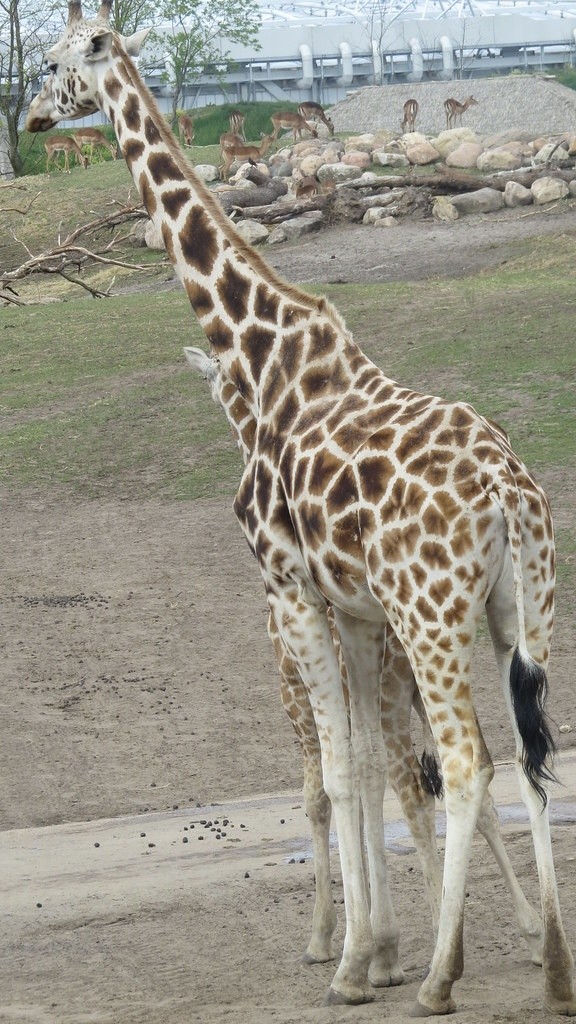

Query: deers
[176,94,480,195]
[45,127,120,175]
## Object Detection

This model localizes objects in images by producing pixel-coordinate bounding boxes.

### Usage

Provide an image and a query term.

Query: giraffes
[20,0,574,1014]
